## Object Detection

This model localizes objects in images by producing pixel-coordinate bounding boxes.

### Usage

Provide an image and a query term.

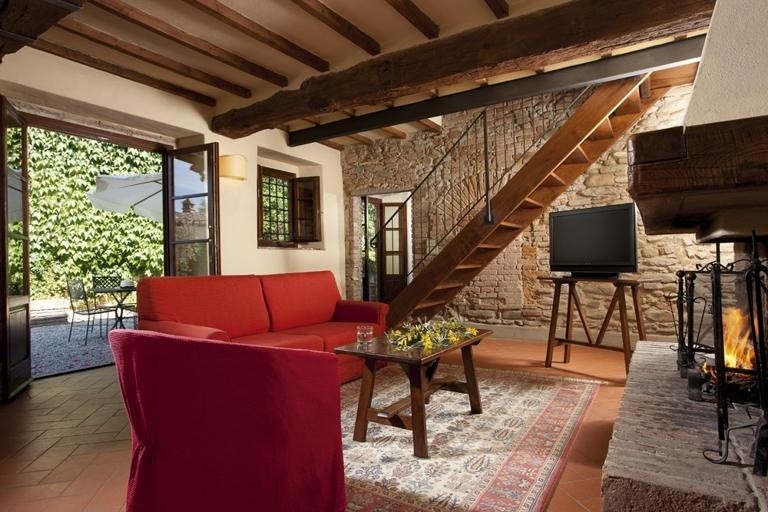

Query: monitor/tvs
[549,203,637,278]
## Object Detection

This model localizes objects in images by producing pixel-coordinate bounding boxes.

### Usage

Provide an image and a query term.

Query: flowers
[383,316,480,352]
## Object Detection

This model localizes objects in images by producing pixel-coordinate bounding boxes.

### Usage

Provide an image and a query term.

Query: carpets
[30,317,135,380]
[339,360,605,511]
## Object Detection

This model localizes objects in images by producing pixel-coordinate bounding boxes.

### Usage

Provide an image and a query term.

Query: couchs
[136,269,392,389]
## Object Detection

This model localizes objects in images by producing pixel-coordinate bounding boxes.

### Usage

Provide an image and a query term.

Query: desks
[93,286,138,329]
[535,274,648,378]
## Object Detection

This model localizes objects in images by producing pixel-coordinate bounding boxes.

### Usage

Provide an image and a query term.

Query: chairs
[91,275,124,339]
[64,279,116,347]
[103,327,351,512]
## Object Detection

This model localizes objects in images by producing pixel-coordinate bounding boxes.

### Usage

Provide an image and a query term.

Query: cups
[357,326,373,343]
